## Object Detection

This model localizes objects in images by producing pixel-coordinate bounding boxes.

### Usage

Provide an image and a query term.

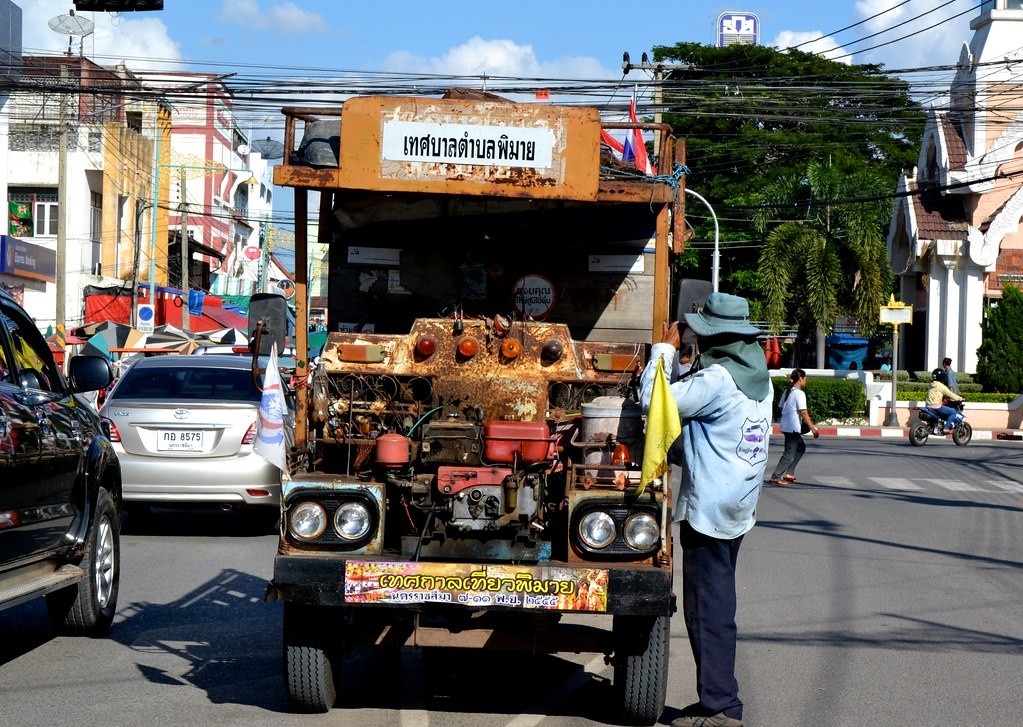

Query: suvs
[0,285,124,638]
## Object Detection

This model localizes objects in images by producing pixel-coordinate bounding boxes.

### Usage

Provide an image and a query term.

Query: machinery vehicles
[242,75,699,727]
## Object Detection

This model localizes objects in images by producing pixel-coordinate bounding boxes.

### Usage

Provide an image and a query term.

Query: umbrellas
[46,319,250,381]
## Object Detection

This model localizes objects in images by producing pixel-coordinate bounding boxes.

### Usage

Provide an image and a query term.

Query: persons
[942,358,958,395]
[925,368,964,434]
[881,359,892,370]
[770,369,819,484]
[639,291,775,727]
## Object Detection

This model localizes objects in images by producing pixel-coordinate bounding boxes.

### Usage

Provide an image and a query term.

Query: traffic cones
[771,336,782,370]
[764,338,772,369]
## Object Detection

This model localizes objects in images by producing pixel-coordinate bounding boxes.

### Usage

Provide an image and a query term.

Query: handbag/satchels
[799,418,811,435]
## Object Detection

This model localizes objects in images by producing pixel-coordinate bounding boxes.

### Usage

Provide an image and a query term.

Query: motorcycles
[908,399,972,447]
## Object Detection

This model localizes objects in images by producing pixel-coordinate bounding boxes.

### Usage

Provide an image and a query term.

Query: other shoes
[682,701,722,717]
[769,479,789,485]
[783,475,799,484]
[943,429,954,435]
[672,711,742,727]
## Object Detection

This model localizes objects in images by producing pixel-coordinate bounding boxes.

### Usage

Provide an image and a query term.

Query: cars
[182,341,300,406]
[95,352,299,519]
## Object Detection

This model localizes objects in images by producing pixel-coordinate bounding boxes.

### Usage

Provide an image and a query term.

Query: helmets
[932,368,945,381]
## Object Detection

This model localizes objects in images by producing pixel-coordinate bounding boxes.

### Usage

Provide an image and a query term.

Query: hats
[684,292,764,337]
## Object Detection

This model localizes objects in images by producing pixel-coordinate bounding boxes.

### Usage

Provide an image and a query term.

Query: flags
[622,96,657,177]
[637,356,682,498]
[255,344,290,475]
